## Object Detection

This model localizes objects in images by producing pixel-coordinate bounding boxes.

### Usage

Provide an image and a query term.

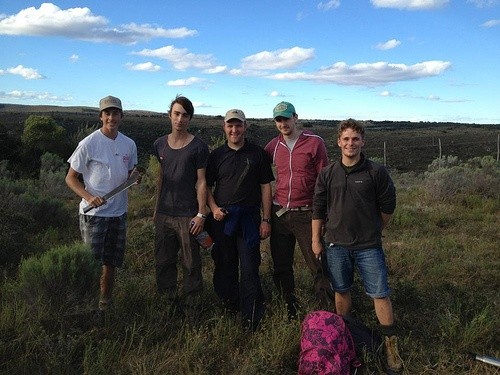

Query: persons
[65,95,141,313]
[205,109,276,330]
[312,119,405,375]
[152,97,209,314]
[263,101,329,323]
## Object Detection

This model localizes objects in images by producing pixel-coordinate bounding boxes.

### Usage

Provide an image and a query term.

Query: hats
[224,110,245,122]
[272,102,297,121]
[99,96,122,111]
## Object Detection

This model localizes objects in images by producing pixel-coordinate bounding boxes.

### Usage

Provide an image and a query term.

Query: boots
[384,335,401,370]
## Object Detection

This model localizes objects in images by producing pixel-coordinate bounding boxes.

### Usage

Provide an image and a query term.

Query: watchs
[262,217,273,225]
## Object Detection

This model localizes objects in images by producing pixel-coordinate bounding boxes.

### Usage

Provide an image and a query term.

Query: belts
[274,205,313,216]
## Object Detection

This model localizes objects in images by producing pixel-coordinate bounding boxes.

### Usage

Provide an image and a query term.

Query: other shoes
[285,303,297,322]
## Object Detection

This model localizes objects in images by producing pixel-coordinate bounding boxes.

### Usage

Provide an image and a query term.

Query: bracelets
[197,214,207,219]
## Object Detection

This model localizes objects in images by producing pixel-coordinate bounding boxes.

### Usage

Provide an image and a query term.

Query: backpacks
[298,311,361,375]
